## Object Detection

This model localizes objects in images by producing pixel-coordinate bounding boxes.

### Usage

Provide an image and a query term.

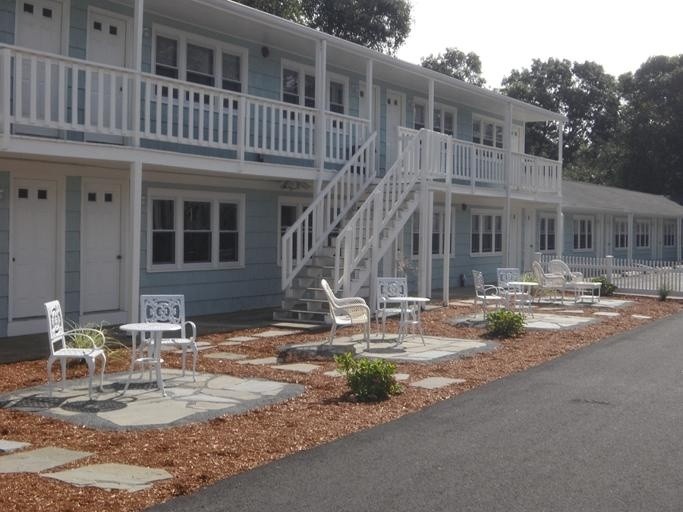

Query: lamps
[283,181,310,190]
[57,163,86,179]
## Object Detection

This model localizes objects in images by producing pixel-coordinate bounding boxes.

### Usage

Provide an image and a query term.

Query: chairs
[546,260,583,304]
[376,277,415,342]
[472,270,509,321]
[321,278,371,351]
[43,300,106,402]
[531,261,566,306]
[496,267,531,317]
[138,294,198,386]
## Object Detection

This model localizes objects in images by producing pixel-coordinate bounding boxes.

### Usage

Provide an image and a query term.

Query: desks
[565,281,602,303]
[117,322,182,398]
[506,281,539,319]
[380,297,430,348]
